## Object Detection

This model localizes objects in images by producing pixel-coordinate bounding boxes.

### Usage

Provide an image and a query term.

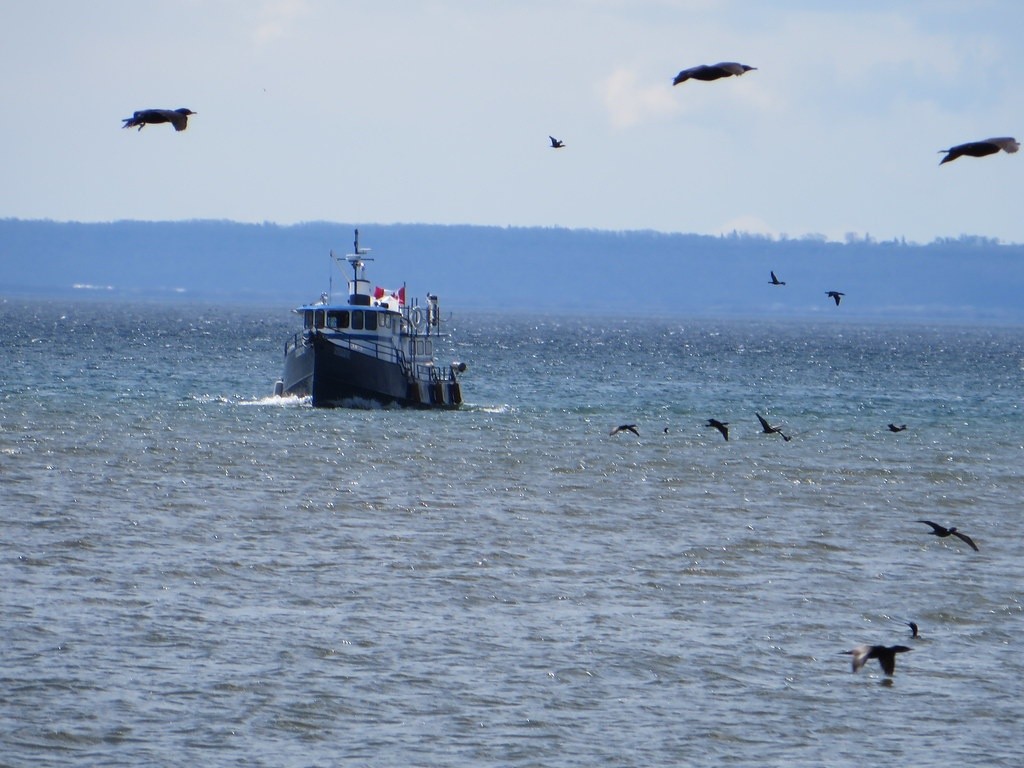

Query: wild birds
[673,62,758,87]
[120,108,197,132]
[704,419,729,442]
[937,137,1021,166]
[903,520,979,551]
[885,423,906,432]
[905,621,922,639]
[772,427,809,441]
[836,645,914,675]
[664,428,669,434]
[549,135,566,148]
[768,271,786,285]
[610,425,640,437]
[825,291,845,306]
[753,411,783,433]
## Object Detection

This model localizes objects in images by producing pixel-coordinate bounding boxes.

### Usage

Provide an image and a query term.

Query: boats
[269,227,467,411]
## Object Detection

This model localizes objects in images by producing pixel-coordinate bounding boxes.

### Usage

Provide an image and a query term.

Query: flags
[374,286,405,305]
